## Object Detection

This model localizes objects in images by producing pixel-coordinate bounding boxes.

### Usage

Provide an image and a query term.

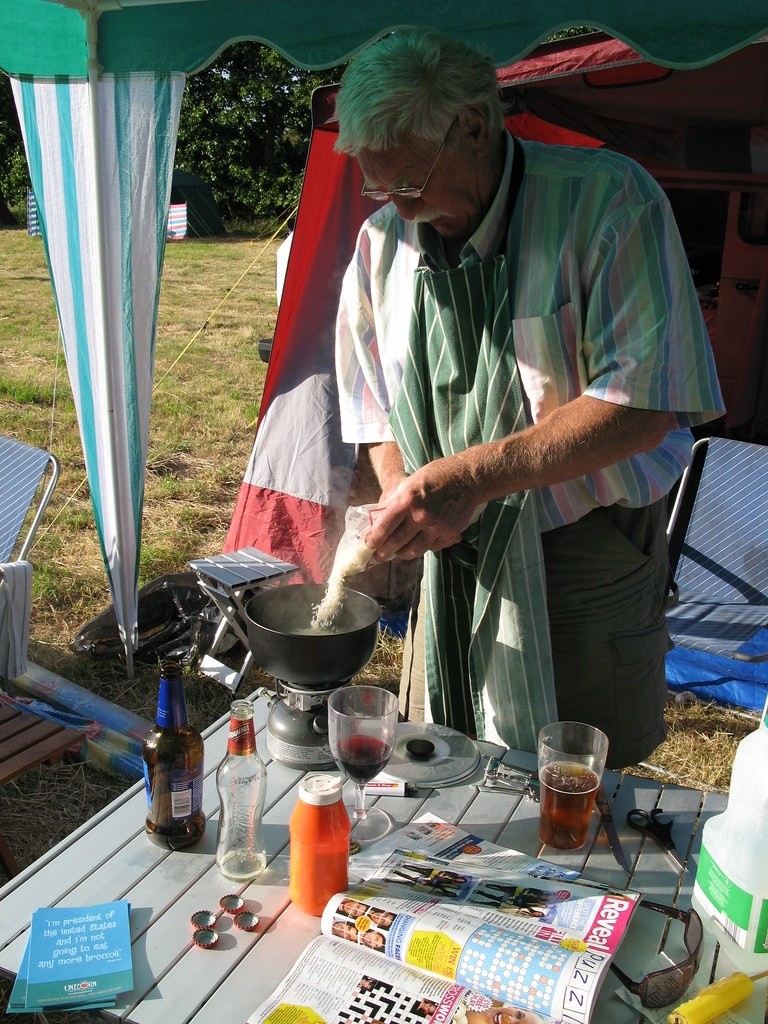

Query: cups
[538,722,609,852]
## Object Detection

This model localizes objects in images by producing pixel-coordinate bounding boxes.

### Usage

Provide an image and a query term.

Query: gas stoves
[267,677,356,770]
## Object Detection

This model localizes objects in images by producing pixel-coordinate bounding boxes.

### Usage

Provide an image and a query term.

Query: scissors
[625,803,691,874]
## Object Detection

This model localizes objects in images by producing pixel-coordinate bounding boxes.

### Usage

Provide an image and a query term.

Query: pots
[244,583,383,686]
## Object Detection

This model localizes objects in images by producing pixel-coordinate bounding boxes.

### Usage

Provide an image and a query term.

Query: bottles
[289,774,352,916]
[216,700,267,881]
[691,711,768,977]
[142,660,206,850]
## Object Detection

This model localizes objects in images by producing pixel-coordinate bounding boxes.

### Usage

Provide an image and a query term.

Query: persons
[330,28,729,771]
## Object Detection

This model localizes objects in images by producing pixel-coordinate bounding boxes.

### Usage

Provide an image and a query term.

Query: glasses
[611,900,705,1010]
[360,114,459,201]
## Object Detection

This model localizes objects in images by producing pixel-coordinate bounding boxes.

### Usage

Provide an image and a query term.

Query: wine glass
[328,685,399,843]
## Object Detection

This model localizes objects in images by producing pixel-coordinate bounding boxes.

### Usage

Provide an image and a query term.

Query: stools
[188,545,299,698]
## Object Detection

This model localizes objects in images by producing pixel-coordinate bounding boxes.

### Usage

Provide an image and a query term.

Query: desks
[0,688,768,1024]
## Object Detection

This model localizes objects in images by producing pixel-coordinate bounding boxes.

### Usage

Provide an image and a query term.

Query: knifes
[595,784,631,878]
